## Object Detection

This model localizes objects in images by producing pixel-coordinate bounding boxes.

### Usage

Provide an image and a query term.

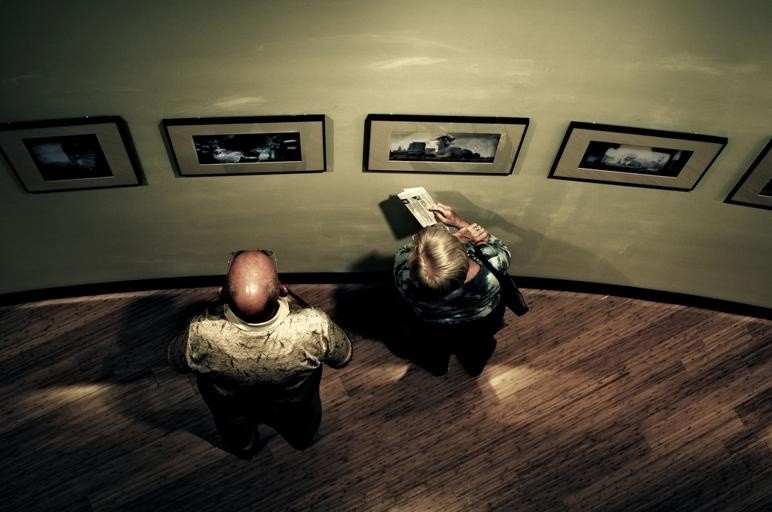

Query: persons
[167,247,355,460]
[390,200,514,379]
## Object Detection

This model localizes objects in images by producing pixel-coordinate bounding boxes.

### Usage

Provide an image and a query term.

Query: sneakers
[429,335,497,379]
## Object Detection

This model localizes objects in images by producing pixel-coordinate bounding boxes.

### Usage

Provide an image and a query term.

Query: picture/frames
[161,112,327,178]
[360,113,529,177]
[547,120,729,192]
[2,115,148,196]
[722,138,772,210]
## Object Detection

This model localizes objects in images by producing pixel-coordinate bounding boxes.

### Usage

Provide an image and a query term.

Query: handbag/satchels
[474,246,529,317]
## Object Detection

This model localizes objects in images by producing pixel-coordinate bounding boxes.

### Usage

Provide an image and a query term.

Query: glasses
[224,247,279,275]
